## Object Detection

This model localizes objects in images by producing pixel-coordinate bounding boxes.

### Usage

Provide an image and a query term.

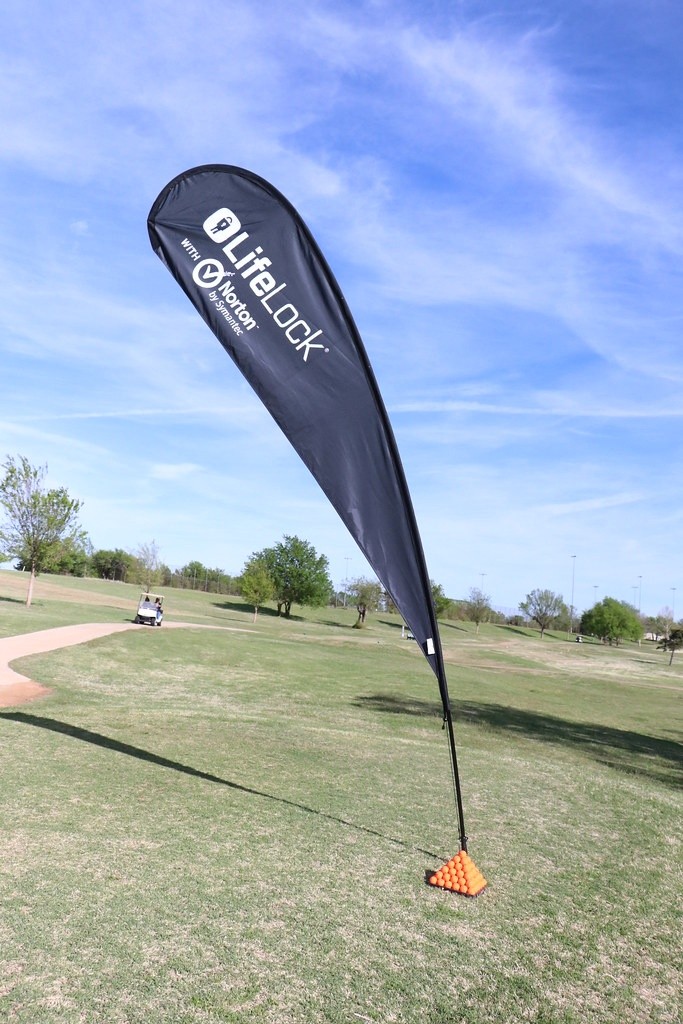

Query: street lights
[570,554,576,636]
[638,574,642,613]
[343,556,354,608]
[632,586,638,606]
[671,588,678,617]
[480,572,488,600]
[593,585,599,603]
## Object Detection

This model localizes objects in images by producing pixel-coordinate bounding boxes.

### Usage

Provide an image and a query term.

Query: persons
[145,597,150,602]
[154,598,163,614]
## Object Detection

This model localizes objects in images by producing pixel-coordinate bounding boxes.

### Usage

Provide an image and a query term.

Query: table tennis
[429,850,487,895]
